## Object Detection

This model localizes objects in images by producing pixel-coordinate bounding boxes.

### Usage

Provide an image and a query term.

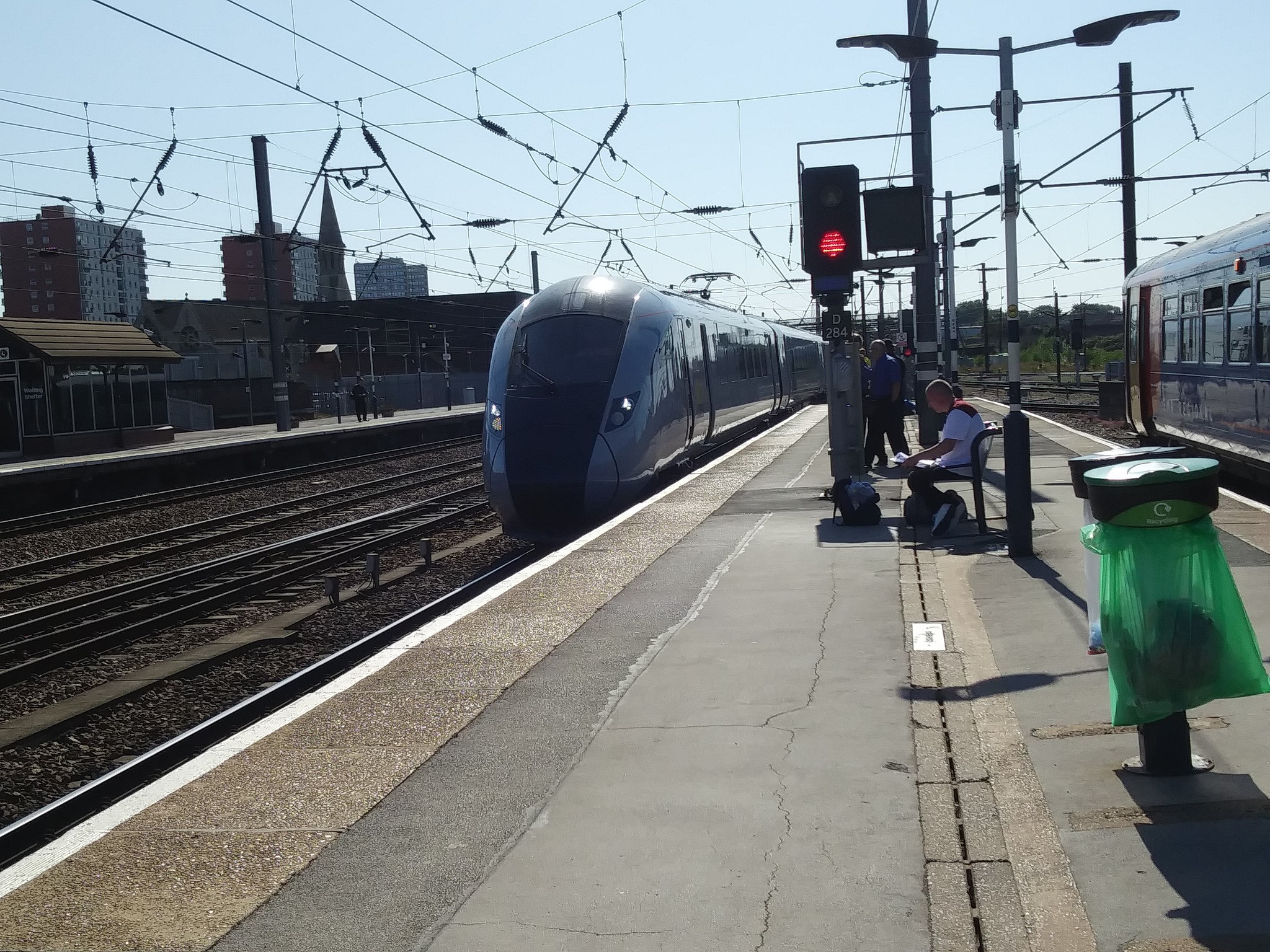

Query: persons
[353,377,369,422]
[901,379,986,536]
[849,333,910,467]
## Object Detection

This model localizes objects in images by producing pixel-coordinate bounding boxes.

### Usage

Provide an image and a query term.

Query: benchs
[930,420,1004,538]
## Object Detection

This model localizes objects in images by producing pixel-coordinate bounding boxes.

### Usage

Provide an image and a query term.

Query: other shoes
[874,455,889,466]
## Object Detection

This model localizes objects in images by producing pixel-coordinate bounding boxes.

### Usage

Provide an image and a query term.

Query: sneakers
[930,501,965,537]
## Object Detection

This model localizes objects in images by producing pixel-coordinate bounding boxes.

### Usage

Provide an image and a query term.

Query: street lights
[837,7,1181,557]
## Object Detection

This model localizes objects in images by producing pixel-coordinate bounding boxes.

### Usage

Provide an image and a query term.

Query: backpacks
[831,479,882,526]
[903,490,968,524]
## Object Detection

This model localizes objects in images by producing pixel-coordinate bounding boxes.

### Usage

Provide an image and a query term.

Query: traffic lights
[800,164,863,274]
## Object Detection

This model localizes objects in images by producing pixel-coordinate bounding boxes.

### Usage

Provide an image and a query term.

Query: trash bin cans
[1065,444,1269,725]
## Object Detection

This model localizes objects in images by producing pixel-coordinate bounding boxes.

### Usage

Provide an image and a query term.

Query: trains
[1123,210,1269,473]
[481,274,830,536]
